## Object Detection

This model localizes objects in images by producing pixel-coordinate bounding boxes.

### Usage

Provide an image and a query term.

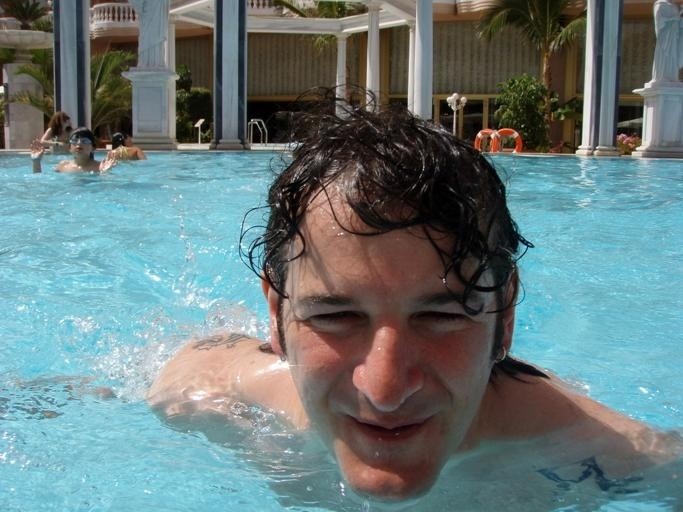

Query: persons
[40,111,74,148]
[29,127,116,176]
[106,131,146,164]
[147,83,683,504]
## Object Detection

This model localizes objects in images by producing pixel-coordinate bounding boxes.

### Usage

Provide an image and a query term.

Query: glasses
[69,136,91,145]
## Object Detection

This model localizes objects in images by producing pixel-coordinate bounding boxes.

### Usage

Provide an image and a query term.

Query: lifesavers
[475,129,501,153]
[498,128,523,153]
